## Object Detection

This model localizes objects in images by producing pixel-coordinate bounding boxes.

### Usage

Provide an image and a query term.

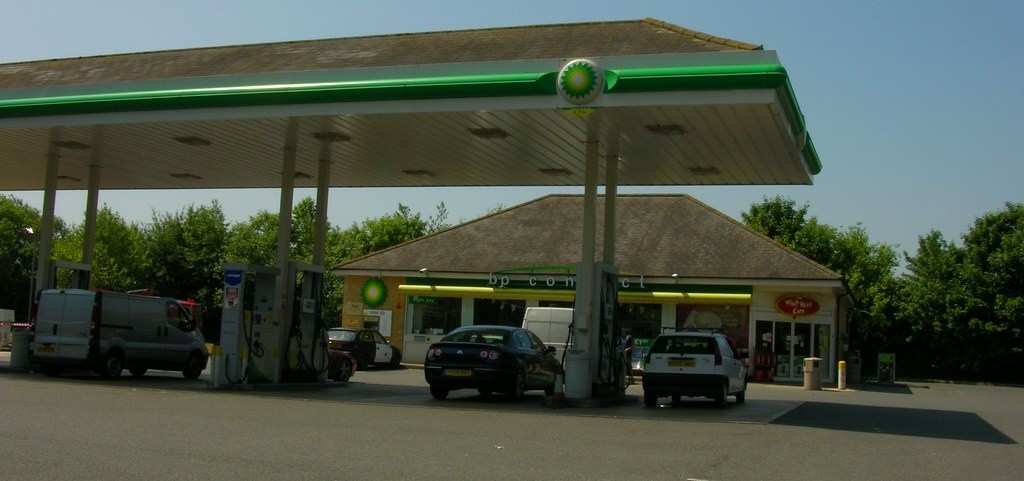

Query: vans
[32,288,209,381]
[522,306,575,370]
[641,327,749,409]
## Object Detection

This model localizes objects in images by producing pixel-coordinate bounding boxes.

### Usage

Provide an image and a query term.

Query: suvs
[327,327,403,371]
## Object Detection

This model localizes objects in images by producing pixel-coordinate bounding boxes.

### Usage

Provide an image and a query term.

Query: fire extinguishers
[756,369,763,383]
[767,369,773,383]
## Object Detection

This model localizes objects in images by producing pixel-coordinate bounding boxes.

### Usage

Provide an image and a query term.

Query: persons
[617,327,637,386]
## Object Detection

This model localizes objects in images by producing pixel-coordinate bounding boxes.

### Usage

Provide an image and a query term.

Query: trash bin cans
[802,357,824,390]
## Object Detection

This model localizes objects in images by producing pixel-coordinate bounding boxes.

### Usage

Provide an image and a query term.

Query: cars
[325,350,357,382]
[424,325,565,403]
